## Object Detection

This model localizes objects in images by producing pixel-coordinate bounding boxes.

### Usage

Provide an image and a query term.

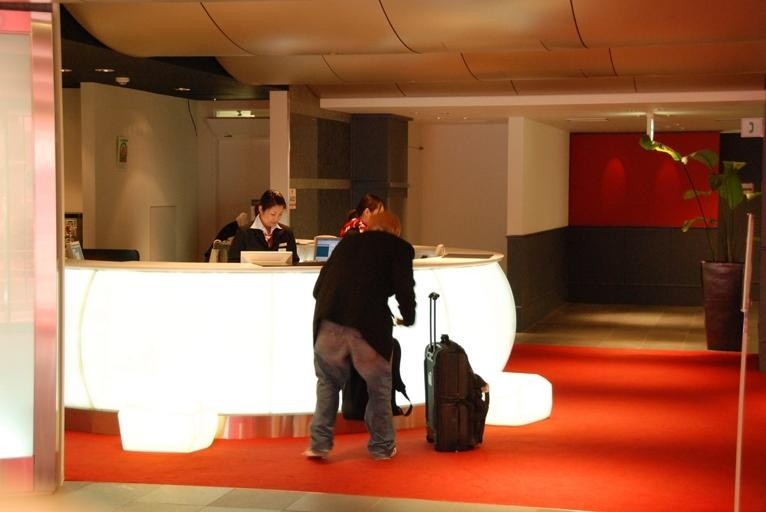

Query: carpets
[64,342,766,512]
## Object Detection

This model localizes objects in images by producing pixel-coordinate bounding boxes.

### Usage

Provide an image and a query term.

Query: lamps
[646,112,656,142]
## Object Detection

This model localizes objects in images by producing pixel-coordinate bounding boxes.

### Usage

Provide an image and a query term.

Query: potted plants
[634,134,762,351]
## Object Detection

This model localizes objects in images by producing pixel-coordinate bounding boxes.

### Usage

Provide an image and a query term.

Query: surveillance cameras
[115,77,130,86]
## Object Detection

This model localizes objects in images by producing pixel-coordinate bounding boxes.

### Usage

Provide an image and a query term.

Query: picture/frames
[64,212,84,250]
[116,135,130,172]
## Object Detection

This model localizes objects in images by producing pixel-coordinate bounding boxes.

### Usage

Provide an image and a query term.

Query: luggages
[424,292,489,452]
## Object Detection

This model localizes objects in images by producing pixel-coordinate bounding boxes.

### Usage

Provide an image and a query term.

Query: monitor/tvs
[314,237,341,263]
[240,251,293,267]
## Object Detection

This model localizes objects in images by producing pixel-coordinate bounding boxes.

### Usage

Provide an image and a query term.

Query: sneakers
[302,447,329,458]
[374,447,397,460]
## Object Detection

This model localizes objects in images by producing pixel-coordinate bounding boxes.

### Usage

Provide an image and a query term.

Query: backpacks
[341,338,412,420]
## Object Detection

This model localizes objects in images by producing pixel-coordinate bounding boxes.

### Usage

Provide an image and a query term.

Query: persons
[340,195,384,237]
[302,210,417,461]
[228,190,300,264]
